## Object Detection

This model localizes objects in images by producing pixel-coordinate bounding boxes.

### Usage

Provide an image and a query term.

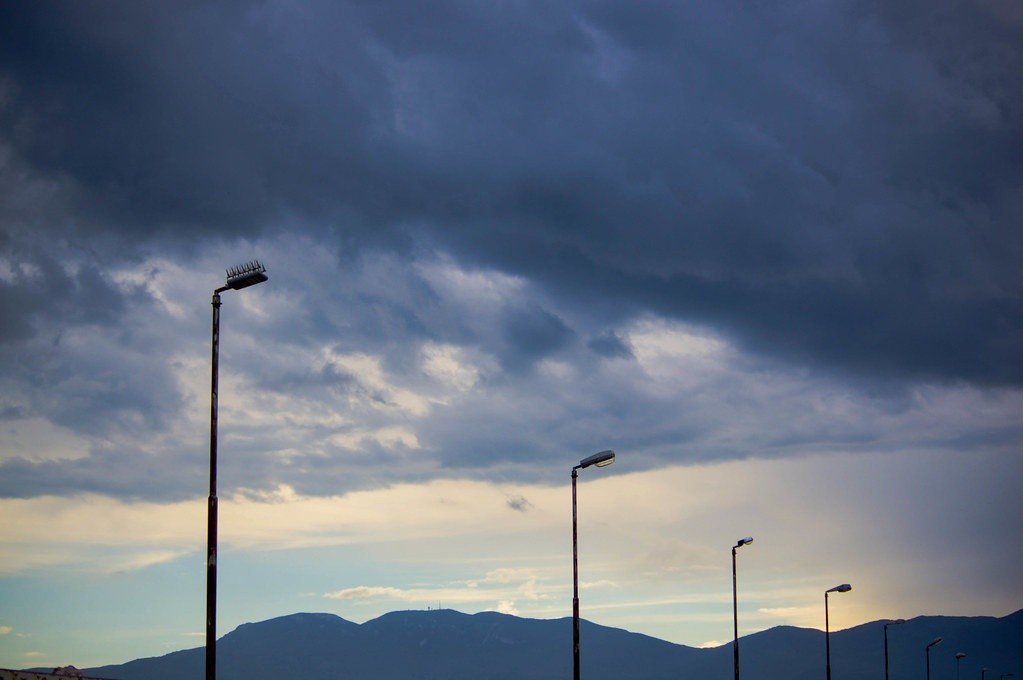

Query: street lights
[956,652,967,680]
[926,637,943,680]
[732,537,753,680]
[573,451,616,680]
[825,584,851,680]
[1001,673,1015,680]
[982,668,992,680]
[884,619,906,680]
[207,259,269,679]
